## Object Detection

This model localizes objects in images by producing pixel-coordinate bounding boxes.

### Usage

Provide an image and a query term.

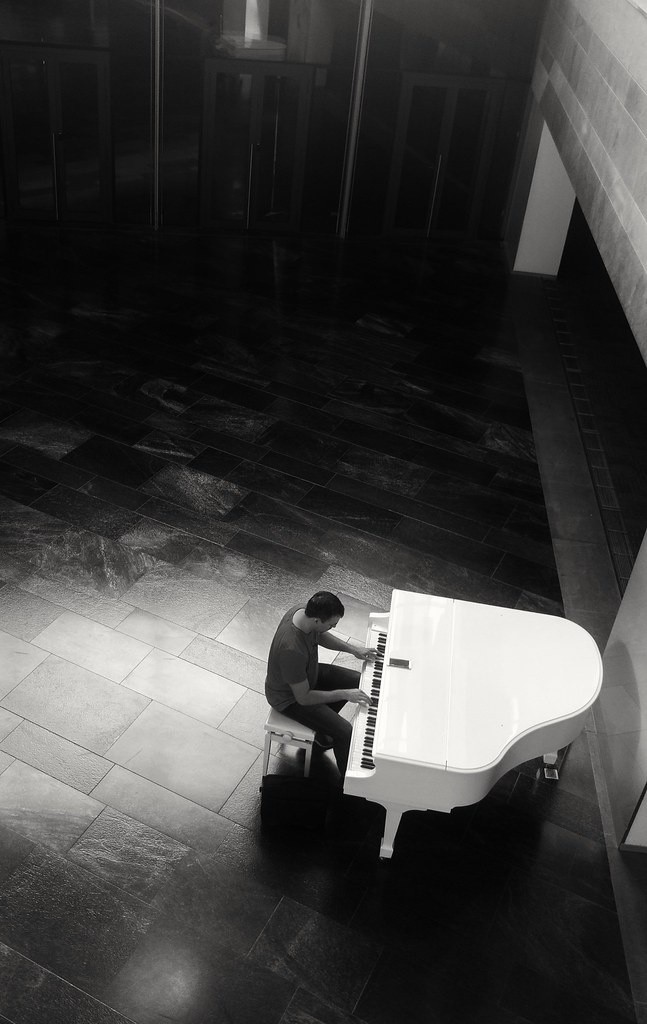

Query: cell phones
[388,657,412,670]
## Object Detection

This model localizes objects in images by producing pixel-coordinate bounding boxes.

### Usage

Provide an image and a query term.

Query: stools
[261,707,316,777]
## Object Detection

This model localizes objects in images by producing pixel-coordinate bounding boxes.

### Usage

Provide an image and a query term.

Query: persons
[265,591,382,777]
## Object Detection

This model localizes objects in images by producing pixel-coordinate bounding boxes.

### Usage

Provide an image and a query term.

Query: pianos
[343,587,603,860]
